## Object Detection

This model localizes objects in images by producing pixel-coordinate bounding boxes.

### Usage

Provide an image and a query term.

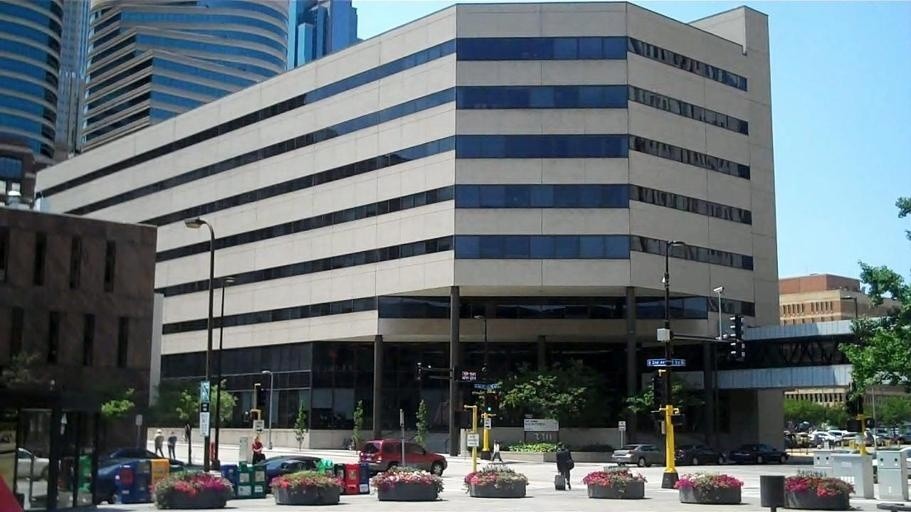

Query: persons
[184,422,191,443]
[153,428,165,458]
[251,436,264,464]
[168,430,177,462]
[492,441,503,462]
[556,442,577,491]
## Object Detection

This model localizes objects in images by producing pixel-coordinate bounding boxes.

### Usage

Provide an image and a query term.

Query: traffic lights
[453,410,472,429]
[846,419,862,432]
[858,394,864,414]
[846,393,855,416]
[730,311,748,362]
[651,411,665,422]
[485,394,499,413]
[243,409,250,423]
[417,368,422,380]
[865,419,874,428]
[668,413,686,427]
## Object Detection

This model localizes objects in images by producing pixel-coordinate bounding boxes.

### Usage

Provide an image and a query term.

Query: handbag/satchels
[567,459,574,470]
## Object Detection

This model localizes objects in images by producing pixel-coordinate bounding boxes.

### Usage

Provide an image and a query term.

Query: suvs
[358,437,447,476]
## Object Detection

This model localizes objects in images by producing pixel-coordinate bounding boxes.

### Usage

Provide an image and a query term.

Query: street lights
[261,368,275,450]
[474,315,488,411]
[842,293,860,331]
[665,241,686,404]
[212,276,274,470]
[185,217,215,472]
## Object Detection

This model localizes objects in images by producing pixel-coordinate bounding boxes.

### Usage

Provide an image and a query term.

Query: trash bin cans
[325,462,370,495]
[220,461,268,498]
[113,459,169,504]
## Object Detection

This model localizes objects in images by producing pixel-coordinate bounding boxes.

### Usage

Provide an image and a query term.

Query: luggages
[555,472,565,490]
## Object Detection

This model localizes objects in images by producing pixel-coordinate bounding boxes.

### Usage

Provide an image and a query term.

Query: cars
[610,444,790,467]
[251,456,322,493]
[784,421,911,449]
[17,447,187,504]
